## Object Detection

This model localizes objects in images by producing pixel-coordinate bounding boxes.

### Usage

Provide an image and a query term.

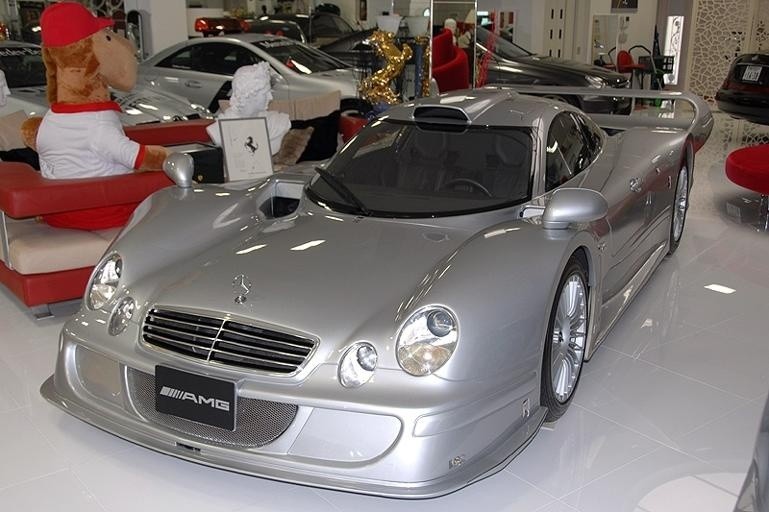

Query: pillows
[218,89,342,165]
[0,108,40,171]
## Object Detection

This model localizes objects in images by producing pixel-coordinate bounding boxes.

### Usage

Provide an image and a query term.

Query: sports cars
[39,78,714,500]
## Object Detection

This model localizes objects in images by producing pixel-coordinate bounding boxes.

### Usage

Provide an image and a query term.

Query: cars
[715,51,769,126]
[138,33,372,119]
[238,12,354,43]
[316,22,633,134]
[0,39,214,153]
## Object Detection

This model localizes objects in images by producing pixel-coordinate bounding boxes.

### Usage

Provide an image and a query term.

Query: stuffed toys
[19,1,170,232]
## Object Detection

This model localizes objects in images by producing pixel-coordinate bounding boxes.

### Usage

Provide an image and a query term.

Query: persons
[205,61,292,156]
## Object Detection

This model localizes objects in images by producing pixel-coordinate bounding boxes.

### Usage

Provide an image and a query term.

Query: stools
[726,142,769,197]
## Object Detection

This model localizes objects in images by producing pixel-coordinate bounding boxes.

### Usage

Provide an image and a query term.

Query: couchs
[122,115,369,184]
[0,156,176,321]
